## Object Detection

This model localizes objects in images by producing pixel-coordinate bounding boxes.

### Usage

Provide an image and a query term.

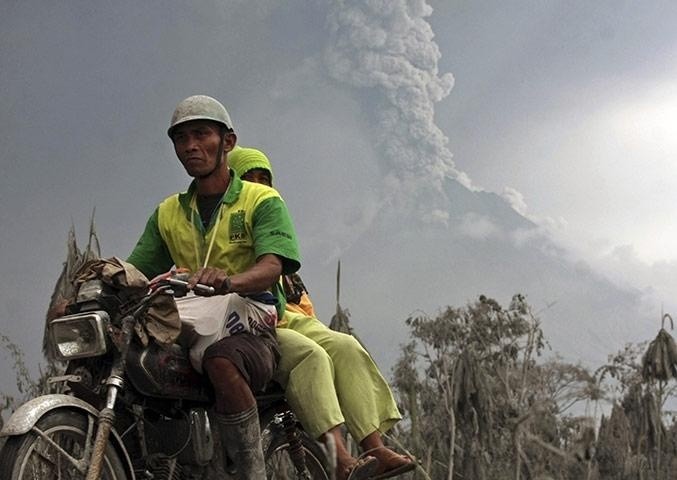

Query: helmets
[168,95,235,139]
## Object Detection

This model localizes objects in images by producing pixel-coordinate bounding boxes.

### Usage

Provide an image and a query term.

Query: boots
[215,401,266,480]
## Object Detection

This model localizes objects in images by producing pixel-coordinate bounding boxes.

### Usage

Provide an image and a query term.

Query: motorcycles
[3,252,335,480]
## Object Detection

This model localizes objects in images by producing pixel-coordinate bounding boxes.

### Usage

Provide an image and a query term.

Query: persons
[226,145,423,479]
[124,96,301,479]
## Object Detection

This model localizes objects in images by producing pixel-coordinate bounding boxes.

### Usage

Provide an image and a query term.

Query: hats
[227,144,273,182]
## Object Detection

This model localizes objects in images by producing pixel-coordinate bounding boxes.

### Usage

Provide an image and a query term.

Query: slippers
[368,455,422,480]
[343,456,378,480]
